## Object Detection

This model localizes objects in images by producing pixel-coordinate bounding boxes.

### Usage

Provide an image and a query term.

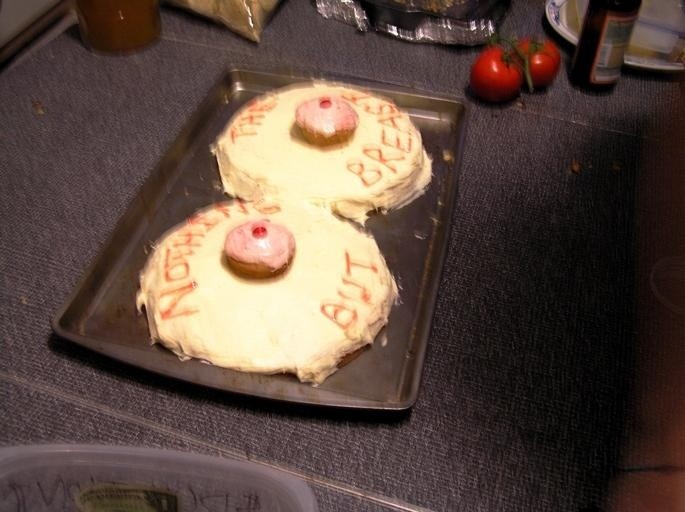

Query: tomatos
[515,34,561,86]
[470,43,524,100]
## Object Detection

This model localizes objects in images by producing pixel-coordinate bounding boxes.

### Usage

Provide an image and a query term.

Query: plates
[543,0,684,73]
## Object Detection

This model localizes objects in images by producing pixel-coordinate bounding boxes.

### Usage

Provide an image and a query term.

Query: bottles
[566,0,643,96]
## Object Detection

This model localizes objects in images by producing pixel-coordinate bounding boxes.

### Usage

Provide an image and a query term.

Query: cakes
[135,198,398,384]
[208,78,430,228]
